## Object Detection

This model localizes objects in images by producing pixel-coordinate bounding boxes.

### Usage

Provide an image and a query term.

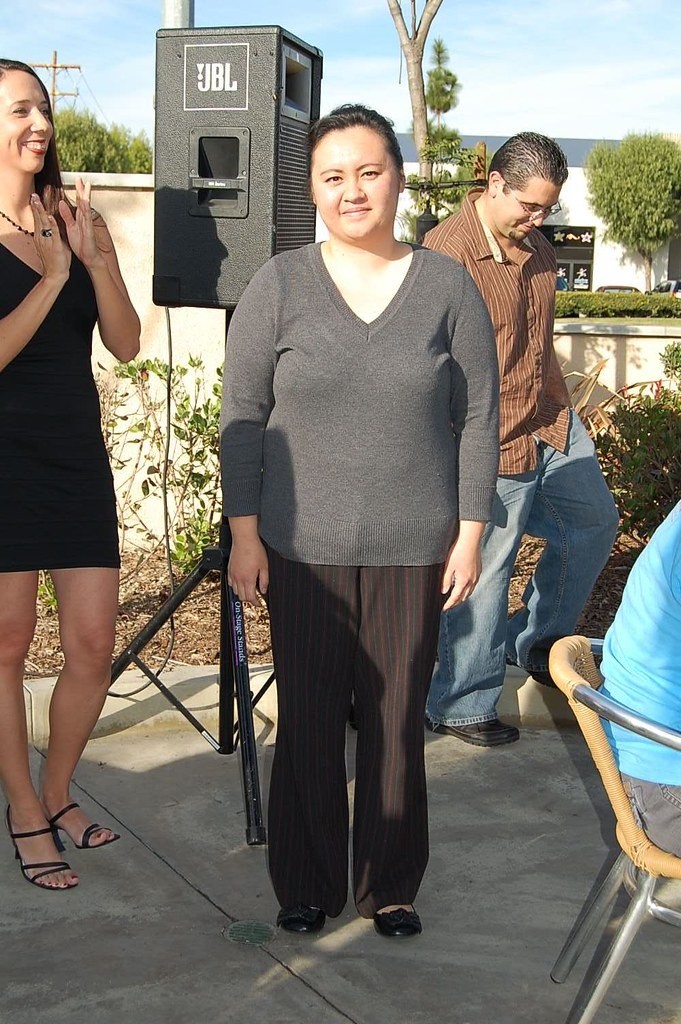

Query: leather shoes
[372,902,422,940]
[277,906,326,936]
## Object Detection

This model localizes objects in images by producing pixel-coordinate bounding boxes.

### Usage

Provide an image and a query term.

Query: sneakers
[504,656,560,690]
[423,714,521,747]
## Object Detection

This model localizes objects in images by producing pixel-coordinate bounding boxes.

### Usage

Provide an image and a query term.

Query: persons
[595,499,681,857]
[417,132,619,747]
[218,103,501,941]
[0,58,141,891]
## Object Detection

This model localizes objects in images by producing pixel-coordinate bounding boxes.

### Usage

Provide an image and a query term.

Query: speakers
[153,24,323,312]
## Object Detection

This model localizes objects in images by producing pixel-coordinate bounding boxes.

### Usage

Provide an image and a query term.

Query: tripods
[111,309,276,846]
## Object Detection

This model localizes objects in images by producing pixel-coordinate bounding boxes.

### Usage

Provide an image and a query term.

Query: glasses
[501,176,561,221]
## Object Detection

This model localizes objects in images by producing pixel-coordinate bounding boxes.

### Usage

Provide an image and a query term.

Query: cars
[555,276,681,300]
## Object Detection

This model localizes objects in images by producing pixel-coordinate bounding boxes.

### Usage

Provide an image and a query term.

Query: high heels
[45,802,121,852]
[5,802,81,890]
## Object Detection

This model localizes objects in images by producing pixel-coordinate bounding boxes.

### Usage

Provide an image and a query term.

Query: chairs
[549,635,681,1023]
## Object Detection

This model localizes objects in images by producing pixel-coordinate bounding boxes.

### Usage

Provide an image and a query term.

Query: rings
[42,229,52,236]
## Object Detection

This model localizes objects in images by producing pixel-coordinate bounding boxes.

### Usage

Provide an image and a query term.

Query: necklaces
[0,211,35,236]
